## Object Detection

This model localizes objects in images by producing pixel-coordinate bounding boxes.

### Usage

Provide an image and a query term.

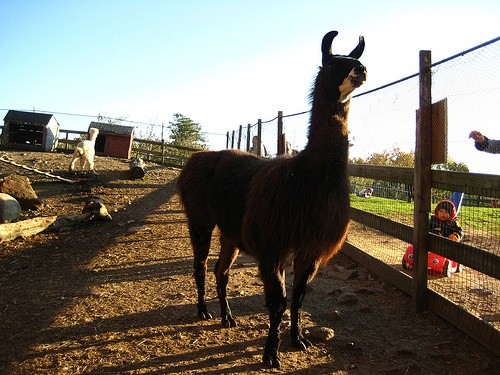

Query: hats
[437,203,452,215]
